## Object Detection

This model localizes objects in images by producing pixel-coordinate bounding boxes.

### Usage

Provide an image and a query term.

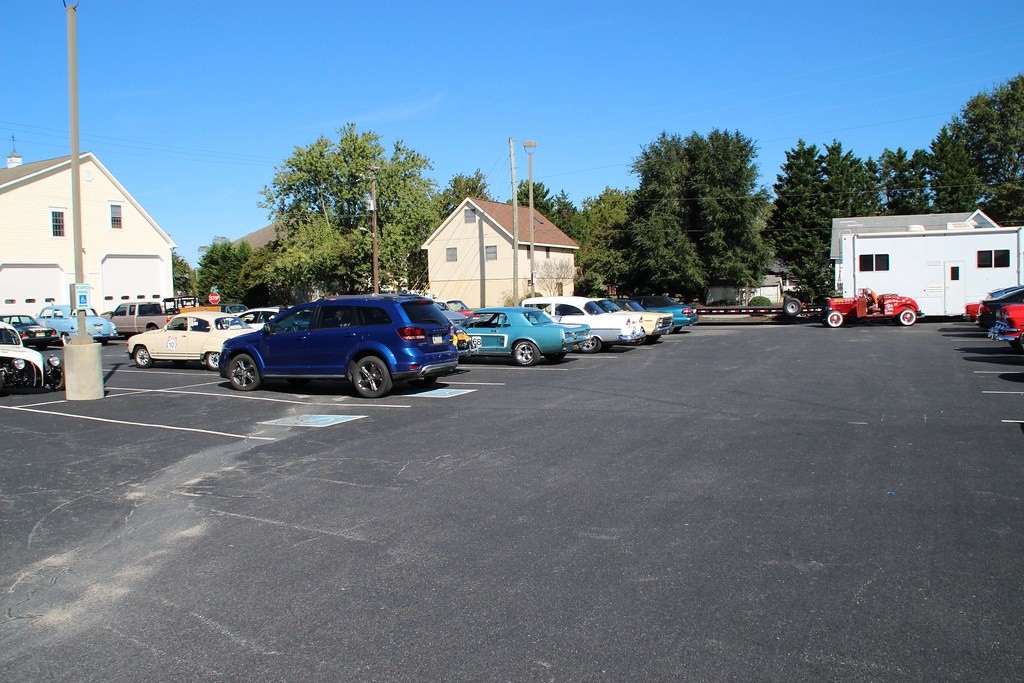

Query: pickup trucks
[109,301,178,341]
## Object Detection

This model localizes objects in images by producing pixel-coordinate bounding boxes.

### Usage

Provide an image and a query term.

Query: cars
[35,305,119,345]
[457,307,591,367]
[0,315,60,352]
[520,296,646,353]
[629,297,699,333]
[0,321,64,394]
[126,310,257,371]
[219,292,460,398]
[432,299,483,375]
[610,298,676,345]
[220,304,297,332]
[965,285,1024,355]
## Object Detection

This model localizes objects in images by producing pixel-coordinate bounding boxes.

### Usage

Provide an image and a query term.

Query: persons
[327,308,351,327]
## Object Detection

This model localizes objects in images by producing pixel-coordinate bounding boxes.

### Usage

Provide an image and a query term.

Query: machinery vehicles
[162,296,250,325]
